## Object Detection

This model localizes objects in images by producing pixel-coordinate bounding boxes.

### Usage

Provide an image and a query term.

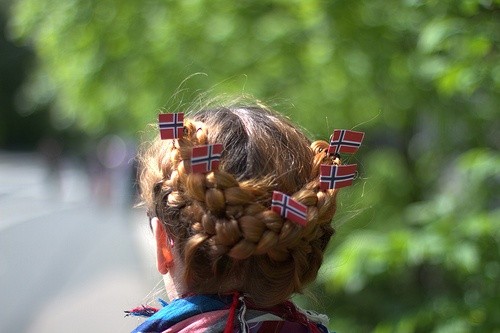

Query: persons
[119,92,355,333]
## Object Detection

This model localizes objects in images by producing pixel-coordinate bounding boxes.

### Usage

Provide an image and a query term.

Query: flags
[327,129,364,155]
[270,189,307,226]
[319,163,357,190]
[191,144,223,173]
[158,112,184,140]
